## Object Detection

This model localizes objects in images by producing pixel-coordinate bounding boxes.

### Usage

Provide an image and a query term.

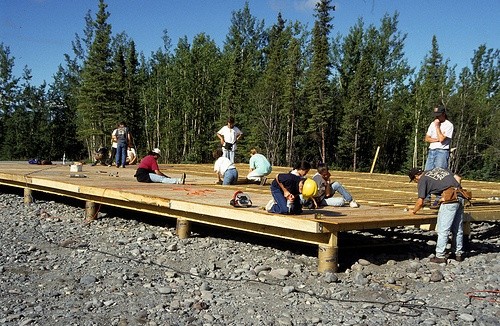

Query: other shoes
[430,257,446,263]
[180,173,186,184]
[446,253,461,261]
[260,177,266,186]
[431,201,438,208]
[349,201,358,208]
[265,200,275,210]
[423,201,430,206]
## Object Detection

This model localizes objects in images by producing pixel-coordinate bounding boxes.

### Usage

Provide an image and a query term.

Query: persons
[213,150,238,185]
[217,117,242,163]
[91,122,137,168]
[135,148,186,184]
[246,147,272,186]
[409,167,472,261]
[266,161,358,214]
[424,106,454,206]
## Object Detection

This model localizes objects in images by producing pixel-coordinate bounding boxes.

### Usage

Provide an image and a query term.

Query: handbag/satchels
[443,189,458,203]
[224,142,233,150]
[460,189,472,200]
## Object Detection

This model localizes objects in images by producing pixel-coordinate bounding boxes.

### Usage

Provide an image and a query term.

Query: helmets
[301,178,317,200]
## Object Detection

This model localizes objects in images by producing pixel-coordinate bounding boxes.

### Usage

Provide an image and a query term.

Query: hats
[409,168,421,183]
[433,105,445,116]
[152,148,163,158]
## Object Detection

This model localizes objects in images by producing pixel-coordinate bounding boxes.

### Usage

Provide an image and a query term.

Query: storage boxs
[70,164,82,172]
